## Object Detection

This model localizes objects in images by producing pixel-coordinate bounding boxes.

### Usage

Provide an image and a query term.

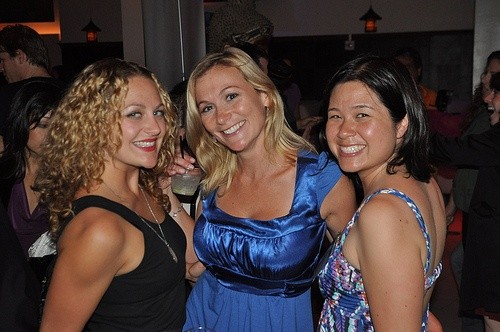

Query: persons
[30,59,189,331]
[0,24,53,85]
[0,77,60,332]
[421,68,500,332]
[157,45,446,331]
[309,52,448,332]
[424,50,500,332]
[164,4,433,178]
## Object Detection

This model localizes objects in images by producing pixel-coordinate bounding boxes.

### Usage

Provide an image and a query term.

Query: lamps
[359,6,383,33]
[81,18,101,43]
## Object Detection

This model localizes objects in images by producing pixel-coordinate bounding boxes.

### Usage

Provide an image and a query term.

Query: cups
[172,168,202,195]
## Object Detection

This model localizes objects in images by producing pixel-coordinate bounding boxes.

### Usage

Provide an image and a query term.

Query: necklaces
[96,177,178,262]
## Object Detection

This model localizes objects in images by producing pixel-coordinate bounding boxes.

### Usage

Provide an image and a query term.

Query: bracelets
[168,202,185,218]
